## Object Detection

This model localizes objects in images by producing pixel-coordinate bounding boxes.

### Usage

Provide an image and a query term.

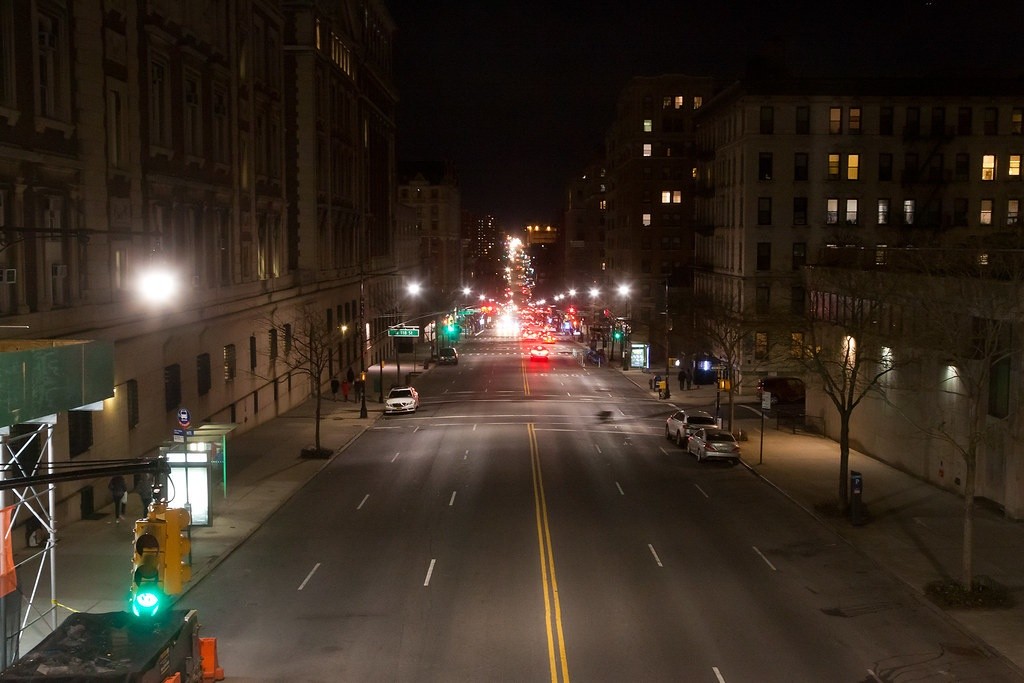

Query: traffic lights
[155,507,193,596]
[447,326,455,332]
[131,519,168,620]
[615,333,621,339]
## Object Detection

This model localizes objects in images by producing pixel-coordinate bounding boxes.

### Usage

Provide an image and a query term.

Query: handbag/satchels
[120,491,128,504]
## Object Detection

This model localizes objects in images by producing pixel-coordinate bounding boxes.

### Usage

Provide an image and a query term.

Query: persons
[347,367,354,391]
[108,475,128,524]
[678,368,686,390]
[686,369,692,390]
[341,379,351,402]
[331,376,339,402]
[353,378,362,403]
[136,473,151,518]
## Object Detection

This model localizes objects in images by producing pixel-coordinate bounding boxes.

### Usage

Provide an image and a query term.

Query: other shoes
[120,515,124,520]
[116,520,119,524]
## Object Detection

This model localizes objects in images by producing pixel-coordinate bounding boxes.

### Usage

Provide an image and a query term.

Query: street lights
[359,270,421,418]
[593,289,599,338]
[568,288,575,317]
[445,286,472,348]
[619,284,629,371]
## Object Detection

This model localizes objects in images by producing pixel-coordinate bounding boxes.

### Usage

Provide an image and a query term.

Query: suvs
[665,409,719,447]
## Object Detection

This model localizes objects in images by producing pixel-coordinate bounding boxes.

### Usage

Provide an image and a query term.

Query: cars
[685,427,741,465]
[542,333,557,344]
[383,387,419,414]
[439,347,459,365]
[530,344,550,361]
